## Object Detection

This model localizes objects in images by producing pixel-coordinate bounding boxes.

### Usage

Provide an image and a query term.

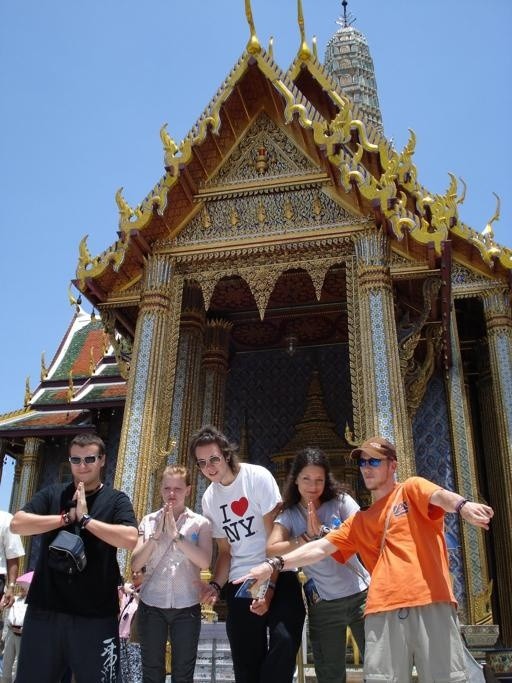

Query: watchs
[79,514,90,525]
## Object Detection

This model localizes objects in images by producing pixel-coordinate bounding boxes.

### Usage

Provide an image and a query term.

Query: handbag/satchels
[130,611,142,643]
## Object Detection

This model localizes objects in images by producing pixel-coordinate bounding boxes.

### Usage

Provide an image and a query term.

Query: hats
[350,437,397,461]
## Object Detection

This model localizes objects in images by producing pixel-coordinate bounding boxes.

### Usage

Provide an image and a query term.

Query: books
[234,579,270,598]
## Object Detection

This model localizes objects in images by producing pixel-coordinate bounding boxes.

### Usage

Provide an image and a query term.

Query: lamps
[284,334,297,357]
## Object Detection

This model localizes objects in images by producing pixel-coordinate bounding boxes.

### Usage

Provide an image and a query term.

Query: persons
[1,511,26,609]
[233,436,493,682]
[188,425,306,682]
[266,447,371,682]
[10,434,139,682]
[1,586,28,682]
[118,565,146,683]
[130,464,212,682]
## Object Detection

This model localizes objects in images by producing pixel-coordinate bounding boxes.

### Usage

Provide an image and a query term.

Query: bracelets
[319,524,330,538]
[7,582,15,588]
[268,582,276,589]
[174,533,184,544]
[455,498,470,516]
[150,534,160,544]
[62,512,74,526]
[296,531,312,545]
[82,517,93,528]
[264,555,285,573]
[209,582,222,596]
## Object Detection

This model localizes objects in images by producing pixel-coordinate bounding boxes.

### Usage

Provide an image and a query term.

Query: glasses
[68,456,99,465]
[357,458,380,466]
[196,456,220,468]
